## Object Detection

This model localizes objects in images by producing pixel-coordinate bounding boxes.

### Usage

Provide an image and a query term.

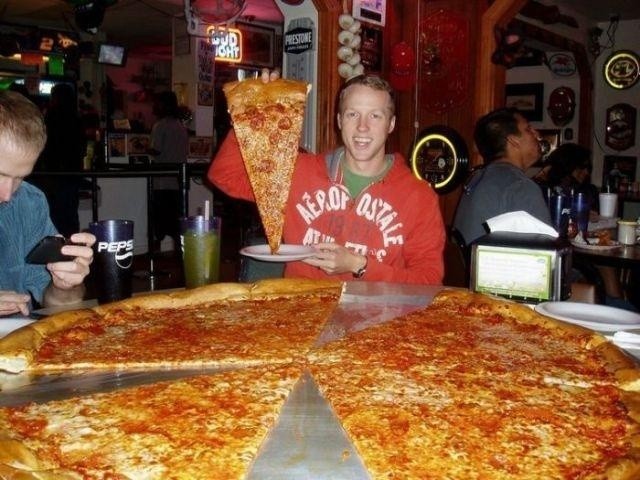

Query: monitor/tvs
[98,44,127,66]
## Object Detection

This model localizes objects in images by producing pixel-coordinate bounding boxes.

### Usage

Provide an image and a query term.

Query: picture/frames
[603,49,640,90]
[506,82,543,122]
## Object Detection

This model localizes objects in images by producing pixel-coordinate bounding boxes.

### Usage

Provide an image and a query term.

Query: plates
[571,238,621,251]
[534,300,640,332]
[239,244,313,262]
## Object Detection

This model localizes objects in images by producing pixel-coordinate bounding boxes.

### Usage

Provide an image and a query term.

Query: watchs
[353,267,366,278]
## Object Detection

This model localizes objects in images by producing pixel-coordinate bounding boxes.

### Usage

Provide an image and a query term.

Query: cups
[547,187,570,247]
[177,216,222,288]
[597,193,618,218]
[569,187,591,234]
[88,218,135,305]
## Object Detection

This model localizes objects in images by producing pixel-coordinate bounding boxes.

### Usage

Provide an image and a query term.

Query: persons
[8,81,190,265]
[450,105,636,312]
[0,87,97,320]
[532,142,622,298]
[208,68,447,288]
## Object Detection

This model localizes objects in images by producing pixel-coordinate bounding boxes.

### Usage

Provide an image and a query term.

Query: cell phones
[25,236,86,265]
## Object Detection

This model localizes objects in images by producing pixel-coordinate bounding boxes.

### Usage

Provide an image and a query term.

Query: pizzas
[0,276,640,480]
[222,76,312,255]
